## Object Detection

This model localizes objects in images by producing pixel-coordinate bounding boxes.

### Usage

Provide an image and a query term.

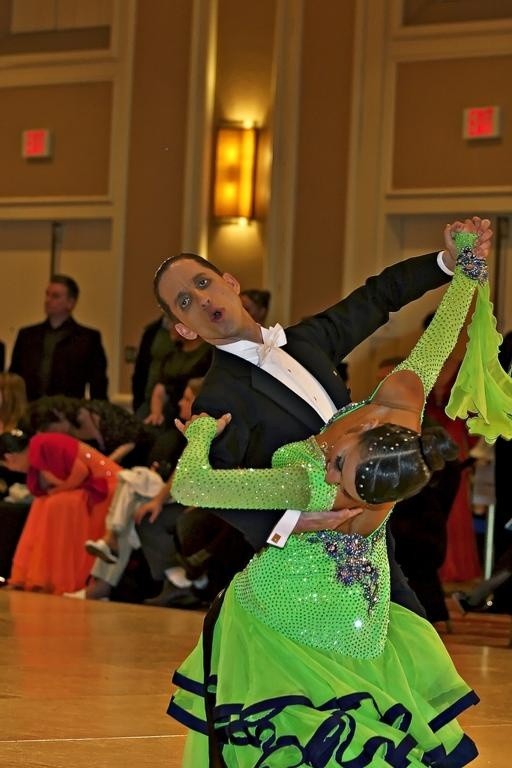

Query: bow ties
[256,318,290,368]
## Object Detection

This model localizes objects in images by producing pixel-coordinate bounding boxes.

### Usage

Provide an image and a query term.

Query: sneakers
[62,587,111,602]
[83,537,120,565]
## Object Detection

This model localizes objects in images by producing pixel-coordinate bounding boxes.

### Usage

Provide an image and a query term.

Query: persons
[238,289,271,326]
[8,275,109,404]
[131,314,182,413]
[154,219,493,623]
[143,322,213,426]
[166,216,486,766]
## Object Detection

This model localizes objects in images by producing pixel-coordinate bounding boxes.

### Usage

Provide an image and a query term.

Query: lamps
[211,120,257,227]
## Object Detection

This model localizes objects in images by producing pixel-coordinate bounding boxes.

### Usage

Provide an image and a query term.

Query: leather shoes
[141,578,214,609]
